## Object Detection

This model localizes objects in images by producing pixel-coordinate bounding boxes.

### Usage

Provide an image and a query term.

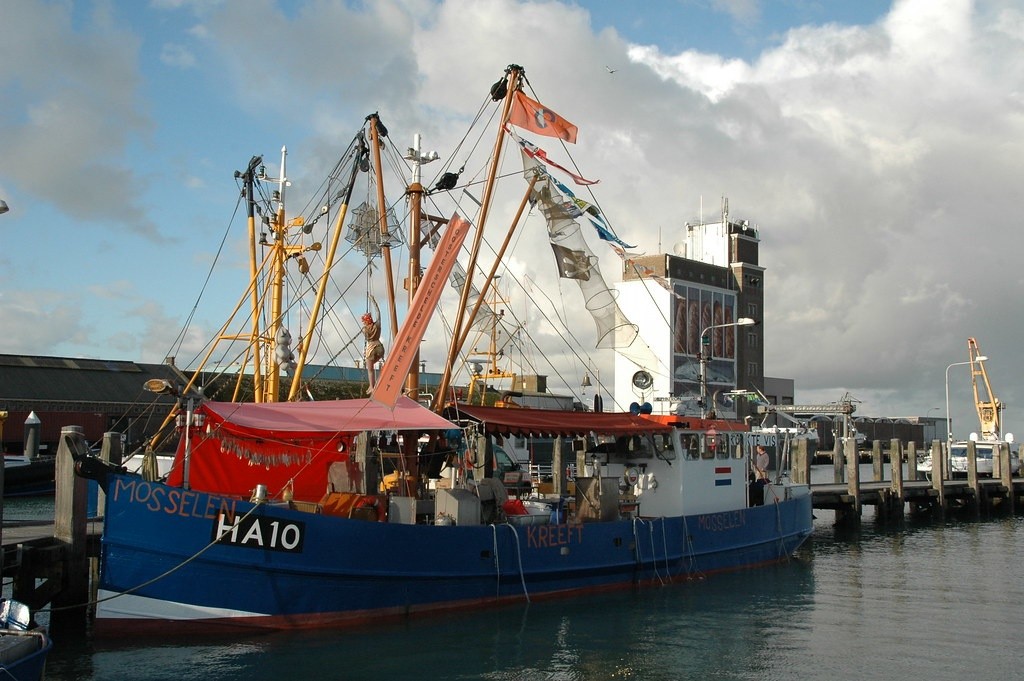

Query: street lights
[926,405,942,444]
[699,317,755,418]
[945,355,989,446]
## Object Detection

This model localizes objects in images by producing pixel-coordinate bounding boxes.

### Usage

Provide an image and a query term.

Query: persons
[371,430,399,454]
[756,445,769,482]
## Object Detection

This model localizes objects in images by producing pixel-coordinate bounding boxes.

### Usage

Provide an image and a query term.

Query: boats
[59,66,813,637]
[0,597,53,681]
[914,442,1022,479]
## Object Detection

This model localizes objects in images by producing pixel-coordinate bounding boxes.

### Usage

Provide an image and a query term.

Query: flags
[506,91,578,144]
[503,126,686,301]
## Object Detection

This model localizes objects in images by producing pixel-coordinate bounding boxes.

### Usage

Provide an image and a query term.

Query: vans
[461,441,532,495]
